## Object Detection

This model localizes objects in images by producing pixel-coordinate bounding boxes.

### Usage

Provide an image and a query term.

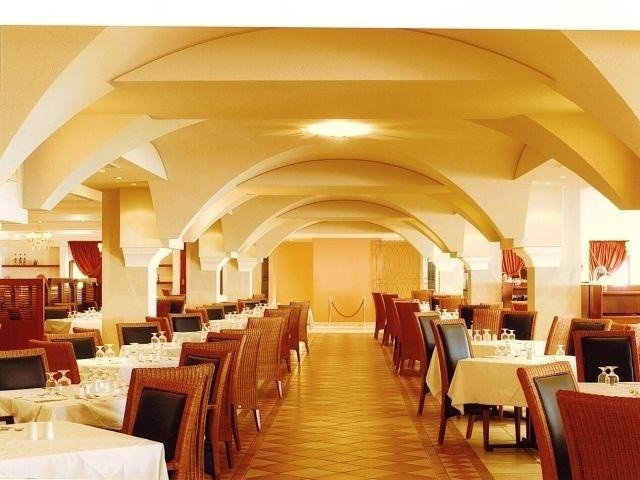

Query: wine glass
[468,328,516,343]
[44,302,275,400]
[414,298,459,319]
[596,365,620,389]
[495,343,567,361]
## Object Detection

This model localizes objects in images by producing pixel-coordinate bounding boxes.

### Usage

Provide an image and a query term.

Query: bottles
[14,252,27,266]
[26,422,55,441]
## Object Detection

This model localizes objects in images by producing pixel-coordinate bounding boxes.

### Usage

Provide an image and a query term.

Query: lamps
[25,220,53,250]
